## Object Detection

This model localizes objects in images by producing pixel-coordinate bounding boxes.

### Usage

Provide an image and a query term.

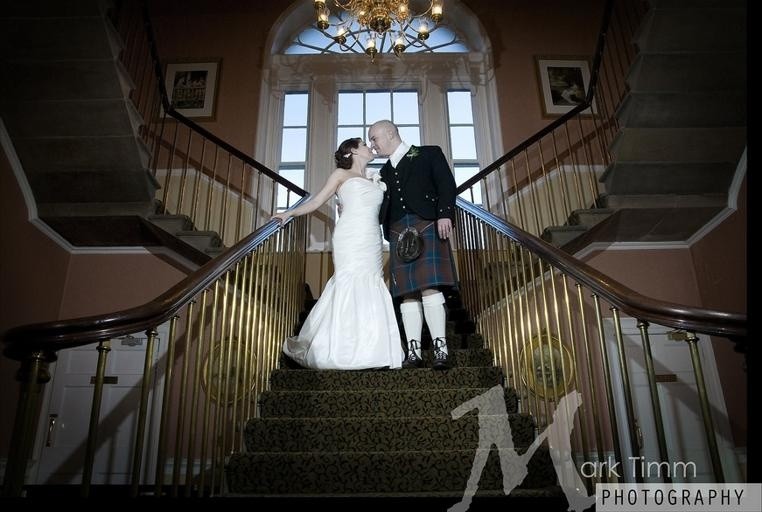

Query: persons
[269,137,406,372]
[368,120,458,371]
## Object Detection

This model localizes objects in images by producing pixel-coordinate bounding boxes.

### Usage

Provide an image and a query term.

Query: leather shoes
[433,352,450,368]
[403,354,424,368]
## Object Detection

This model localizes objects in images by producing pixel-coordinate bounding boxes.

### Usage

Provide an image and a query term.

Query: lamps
[314,0,445,66]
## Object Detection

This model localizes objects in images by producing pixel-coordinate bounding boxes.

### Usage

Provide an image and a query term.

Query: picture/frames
[530,53,607,120]
[149,55,223,124]
[521,327,576,402]
[200,331,262,408]
